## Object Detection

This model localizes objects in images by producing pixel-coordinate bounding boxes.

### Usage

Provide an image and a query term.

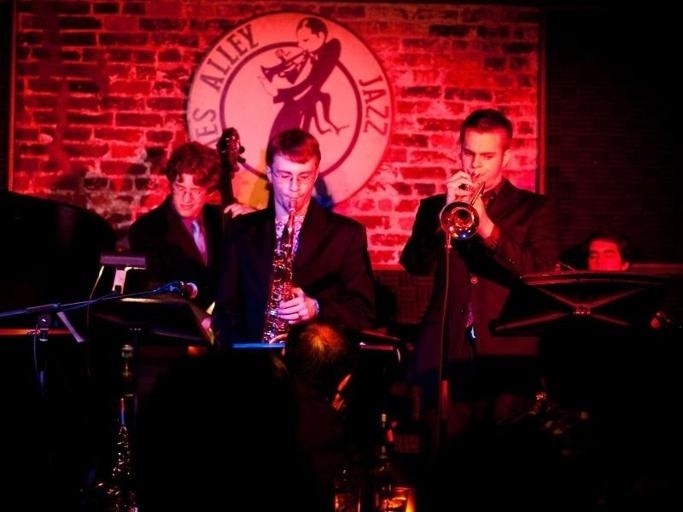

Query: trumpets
[438,169,486,240]
[259,51,308,81]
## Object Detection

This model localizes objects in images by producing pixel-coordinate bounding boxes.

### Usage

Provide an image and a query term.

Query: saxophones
[263,198,296,371]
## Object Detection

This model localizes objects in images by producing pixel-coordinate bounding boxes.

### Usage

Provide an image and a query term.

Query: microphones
[151,280,199,298]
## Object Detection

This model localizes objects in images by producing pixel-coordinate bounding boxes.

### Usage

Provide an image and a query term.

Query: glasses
[170,182,210,198]
[271,170,317,184]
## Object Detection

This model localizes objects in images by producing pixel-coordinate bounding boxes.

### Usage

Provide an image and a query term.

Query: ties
[190,219,209,266]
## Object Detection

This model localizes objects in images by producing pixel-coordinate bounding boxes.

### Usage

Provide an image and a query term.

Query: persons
[402,110,557,421]
[585,232,634,276]
[126,139,258,348]
[236,319,365,511]
[463,314,651,511]
[214,128,378,391]
